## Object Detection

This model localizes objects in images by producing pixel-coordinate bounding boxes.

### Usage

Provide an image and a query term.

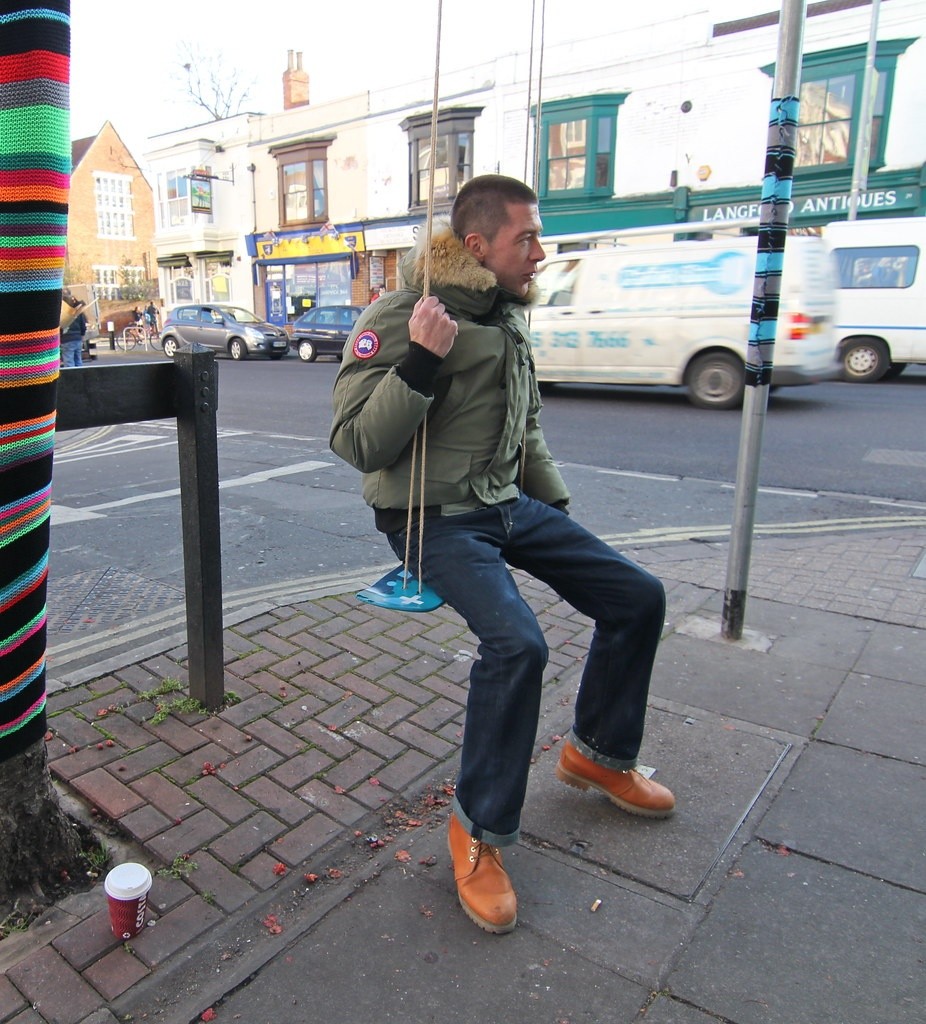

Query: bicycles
[115,322,162,351]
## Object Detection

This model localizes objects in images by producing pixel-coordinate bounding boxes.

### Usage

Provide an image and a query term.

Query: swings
[353,1,551,614]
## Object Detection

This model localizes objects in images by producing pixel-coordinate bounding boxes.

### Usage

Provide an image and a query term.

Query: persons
[60,288,85,367]
[328,174,678,933]
[132,301,160,334]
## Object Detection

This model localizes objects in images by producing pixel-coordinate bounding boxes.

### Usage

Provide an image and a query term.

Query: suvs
[163,302,288,360]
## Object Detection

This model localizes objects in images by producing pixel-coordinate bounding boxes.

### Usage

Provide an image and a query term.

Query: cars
[289,304,365,362]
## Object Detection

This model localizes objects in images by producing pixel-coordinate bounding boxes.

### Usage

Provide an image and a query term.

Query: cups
[103,862,153,941]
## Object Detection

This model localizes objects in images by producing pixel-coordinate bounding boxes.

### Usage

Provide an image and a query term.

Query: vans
[518,215,926,409]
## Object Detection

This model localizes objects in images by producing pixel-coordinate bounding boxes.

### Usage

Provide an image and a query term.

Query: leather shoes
[447,812,517,934]
[554,739,675,818]
